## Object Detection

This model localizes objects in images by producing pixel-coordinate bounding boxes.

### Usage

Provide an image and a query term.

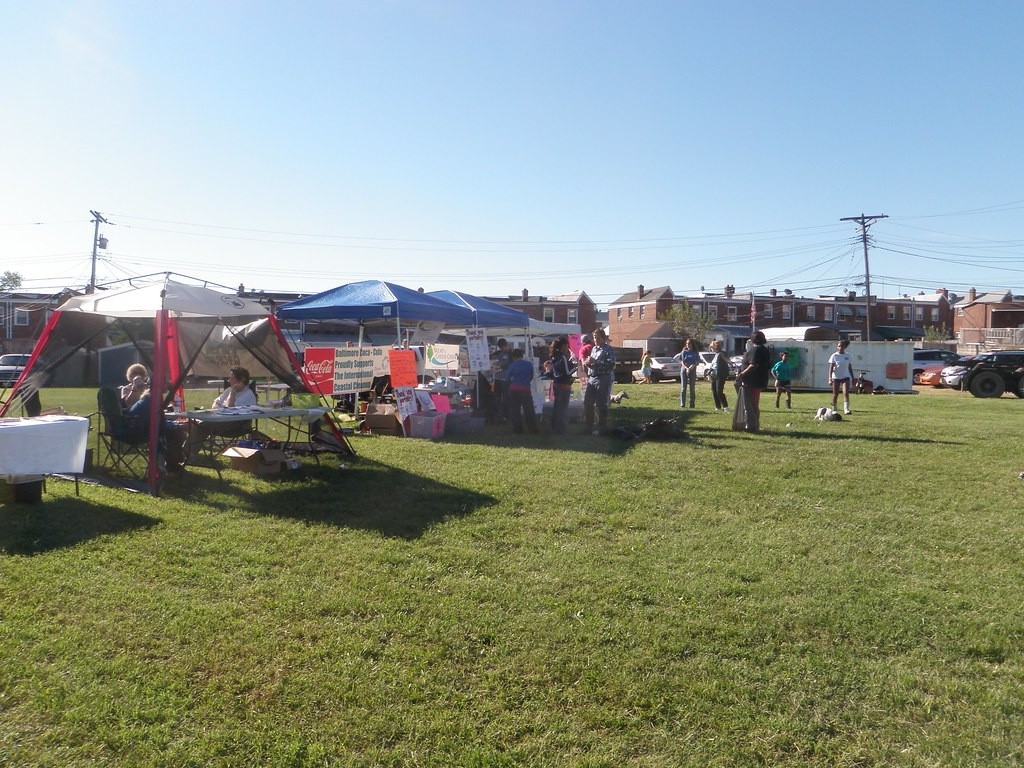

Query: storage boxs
[5,472,44,503]
[222,446,301,475]
[364,404,485,439]
[291,392,320,409]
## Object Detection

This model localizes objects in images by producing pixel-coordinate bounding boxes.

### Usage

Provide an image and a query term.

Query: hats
[581,334,590,342]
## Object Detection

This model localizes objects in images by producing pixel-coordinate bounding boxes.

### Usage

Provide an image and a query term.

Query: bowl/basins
[428,384,442,389]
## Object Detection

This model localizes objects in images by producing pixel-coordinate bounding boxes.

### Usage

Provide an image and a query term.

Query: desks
[164,406,333,481]
[0,415,88,496]
[415,384,474,412]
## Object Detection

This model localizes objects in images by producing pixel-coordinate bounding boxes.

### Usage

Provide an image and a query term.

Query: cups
[588,356,593,359]
[573,362,577,367]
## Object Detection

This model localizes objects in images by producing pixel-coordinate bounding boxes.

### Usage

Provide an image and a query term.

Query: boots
[831,402,837,411]
[843,401,850,414]
[786,400,791,408]
[776,400,779,408]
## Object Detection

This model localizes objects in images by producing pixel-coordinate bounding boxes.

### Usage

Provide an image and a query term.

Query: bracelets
[131,389,137,392]
[829,378,832,380]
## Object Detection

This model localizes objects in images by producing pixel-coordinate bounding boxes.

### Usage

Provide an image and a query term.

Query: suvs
[359,345,438,402]
[913,347,1024,399]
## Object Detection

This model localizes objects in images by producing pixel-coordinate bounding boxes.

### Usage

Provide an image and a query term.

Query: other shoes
[716,408,723,411]
[158,456,167,472]
[724,406,730,413]
[169,463,187,477]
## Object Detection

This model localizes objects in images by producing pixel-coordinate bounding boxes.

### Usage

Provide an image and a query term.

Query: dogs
[814,407,842,422]
[610,390,629,406]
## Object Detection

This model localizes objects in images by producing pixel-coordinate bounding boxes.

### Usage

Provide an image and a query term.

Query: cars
[629,356,682,384]
[0,354,41,388]
[672,351,745,382]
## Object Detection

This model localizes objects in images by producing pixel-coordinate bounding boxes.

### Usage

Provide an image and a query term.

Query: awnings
[873,327,945,339]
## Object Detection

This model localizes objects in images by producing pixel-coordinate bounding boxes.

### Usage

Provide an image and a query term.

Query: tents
[0,268,358,496]
[273,279,480,420]
[406,317,586,393]
[360,289,543,421]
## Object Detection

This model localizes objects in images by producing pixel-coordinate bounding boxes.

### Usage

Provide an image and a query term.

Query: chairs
[96,387,184,483]
[194,380,258,459]
[19,385,95,431]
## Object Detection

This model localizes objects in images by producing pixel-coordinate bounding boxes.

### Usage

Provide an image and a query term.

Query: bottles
[174,391,182,413]
[786,422,793,426]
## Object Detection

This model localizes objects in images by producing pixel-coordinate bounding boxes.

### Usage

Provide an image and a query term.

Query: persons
[121,363,149,407]
[577,327,616,436]
[828,339,856,414]
[638,349,653,384]
[130,388,187,477]
[734,331,770,431]
[770,351,792,408]
[707,342,733,413]
[673,338,706,408]
[183,367,256,462]
[489,338,512,422]
[543,360,554,379]
[346,341,354,347]
[579,332,599,425]
[503,349,540,434]
[402,339,419,371]
[549,335,579,436]
